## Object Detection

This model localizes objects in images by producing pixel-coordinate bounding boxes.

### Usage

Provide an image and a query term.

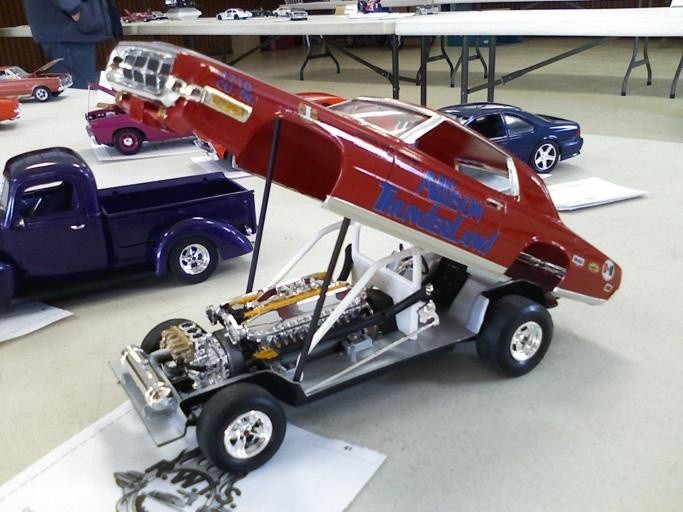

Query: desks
[0,6,682,108]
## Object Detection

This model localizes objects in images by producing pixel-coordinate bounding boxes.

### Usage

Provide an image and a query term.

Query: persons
[24,0,115,91]
